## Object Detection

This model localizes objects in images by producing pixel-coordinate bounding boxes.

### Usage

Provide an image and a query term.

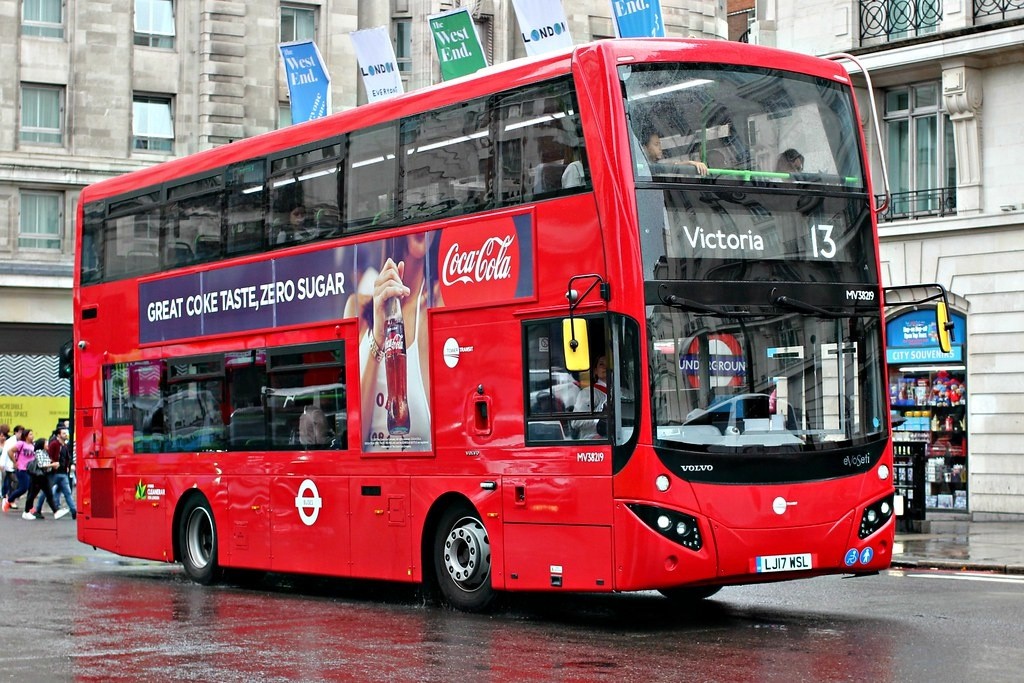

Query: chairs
[109,163,567,277]
[230,407,264,446]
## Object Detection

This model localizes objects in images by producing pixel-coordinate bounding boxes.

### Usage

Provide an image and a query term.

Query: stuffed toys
[928,370,966,407]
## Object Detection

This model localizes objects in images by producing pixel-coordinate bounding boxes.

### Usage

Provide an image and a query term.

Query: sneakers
[22,511,36,520]
[54,509,70,519]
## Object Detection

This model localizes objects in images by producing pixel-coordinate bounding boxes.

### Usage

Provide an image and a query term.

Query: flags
[612,0,666,40]
[510,1,574,57]
[347,24,405,104]
[426,5,489,82]
[280,37,333,127]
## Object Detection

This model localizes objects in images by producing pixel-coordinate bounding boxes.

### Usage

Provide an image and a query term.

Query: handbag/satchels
[26,459,46,478]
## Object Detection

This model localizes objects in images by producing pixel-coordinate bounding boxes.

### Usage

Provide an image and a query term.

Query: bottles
[384,297,410,436]
[946,416,953,431]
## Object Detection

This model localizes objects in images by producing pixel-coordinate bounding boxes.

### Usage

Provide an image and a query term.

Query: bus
[72,36,955,613]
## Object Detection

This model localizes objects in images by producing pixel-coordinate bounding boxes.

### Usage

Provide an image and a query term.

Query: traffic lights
[58,338,73,378]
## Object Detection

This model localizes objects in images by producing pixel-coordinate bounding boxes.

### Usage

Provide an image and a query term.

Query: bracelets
[369,335,385,363]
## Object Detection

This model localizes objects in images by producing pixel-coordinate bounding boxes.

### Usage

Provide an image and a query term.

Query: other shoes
[33,512,44,519]
[29,507,36,514]
[7,498,18,509]
[72,511,76,519]
[2,498,10,512]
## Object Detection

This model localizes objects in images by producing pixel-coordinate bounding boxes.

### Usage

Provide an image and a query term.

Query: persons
[639,128,727,177]
[561,150,587,191]
[343,227,448,454]
[0,418,76,520]
[275,200,318,245]
[530,391,570,440]
[570,352,609,440]
[773,148,804,174]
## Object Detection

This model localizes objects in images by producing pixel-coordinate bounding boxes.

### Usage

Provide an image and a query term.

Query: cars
[526,364,581,413]
